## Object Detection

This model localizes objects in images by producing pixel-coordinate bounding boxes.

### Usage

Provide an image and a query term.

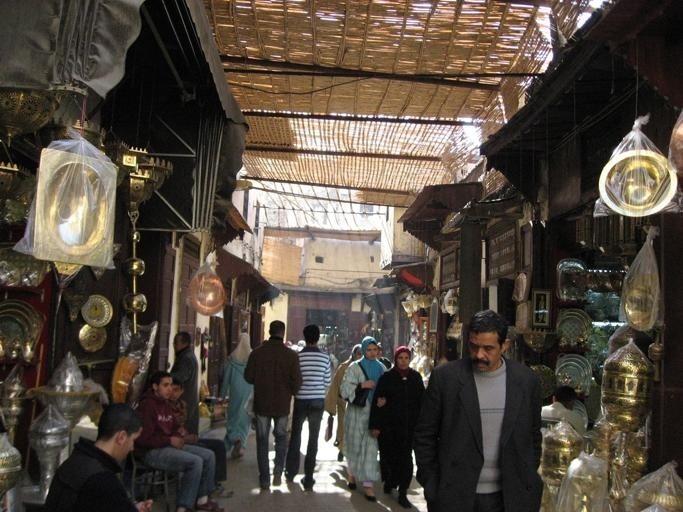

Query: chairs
[129,451,182,512]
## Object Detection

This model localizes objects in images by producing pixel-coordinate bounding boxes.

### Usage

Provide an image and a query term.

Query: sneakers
[177,440,412,512]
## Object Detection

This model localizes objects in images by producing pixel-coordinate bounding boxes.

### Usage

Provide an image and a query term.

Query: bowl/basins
[598,150,678,218]
[189,272,226,315]
[32,147,118,269]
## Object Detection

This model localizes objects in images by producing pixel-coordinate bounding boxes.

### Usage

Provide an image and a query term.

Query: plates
[79,324,107,352]
[556,308,592,347]
[0,299,43,355]
[81,294,113,328]
[555,354,592,393]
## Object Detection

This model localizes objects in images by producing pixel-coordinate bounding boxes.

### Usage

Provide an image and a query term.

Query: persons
[134,320,425,512]
[415,308,543,512]
[541,386,586,434]
[41,403,153,512]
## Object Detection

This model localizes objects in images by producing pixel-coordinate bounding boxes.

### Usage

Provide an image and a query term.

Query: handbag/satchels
[325,415,334,441]
[339,387,369,407]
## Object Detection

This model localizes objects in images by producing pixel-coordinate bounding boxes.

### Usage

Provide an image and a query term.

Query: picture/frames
[531,287,553,329]
